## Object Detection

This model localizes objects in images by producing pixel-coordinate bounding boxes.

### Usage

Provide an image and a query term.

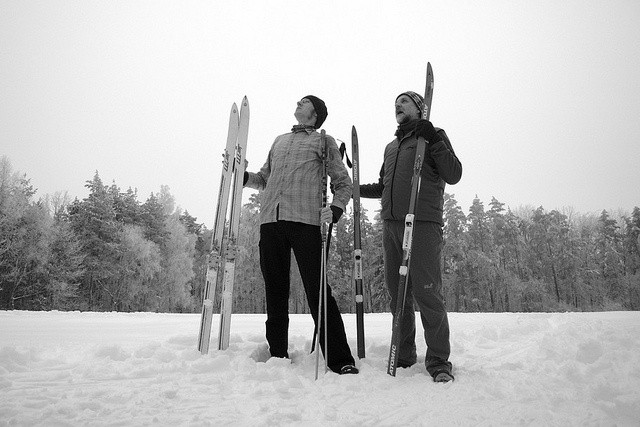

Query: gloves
[415,119,443,147]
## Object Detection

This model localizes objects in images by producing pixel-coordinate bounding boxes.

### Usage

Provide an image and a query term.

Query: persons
[353,92,463,384]
[228,96,360,375]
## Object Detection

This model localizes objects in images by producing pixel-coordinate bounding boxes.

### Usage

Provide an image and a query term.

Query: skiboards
[351,62,435,377]
[198,95,249,355]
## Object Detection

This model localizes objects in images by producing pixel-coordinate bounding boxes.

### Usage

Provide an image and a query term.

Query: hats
[302,95,328,128]
[396,91,425,118]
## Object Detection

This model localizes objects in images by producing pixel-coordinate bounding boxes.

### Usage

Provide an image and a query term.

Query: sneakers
[433,369,455,382]
[339,362,359,373]
